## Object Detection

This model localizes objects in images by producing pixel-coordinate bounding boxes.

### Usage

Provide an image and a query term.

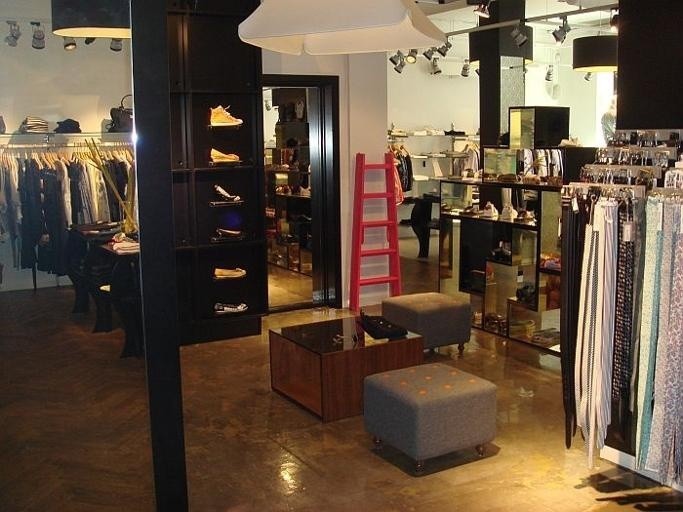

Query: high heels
[213,185,241,202]
[210,148,240,163]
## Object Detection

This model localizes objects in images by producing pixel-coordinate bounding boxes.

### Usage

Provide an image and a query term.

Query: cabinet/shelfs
[438,105,599,356]
[387,126,480,227]
[171,85,270,317]
[263,120,312,278]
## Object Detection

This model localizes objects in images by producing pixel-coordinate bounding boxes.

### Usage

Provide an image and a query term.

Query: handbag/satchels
[522,174,541,185]
[538,257,562,272]
[278,101,296,122]
[515,275,565,311]
[487,240,513,265]
[482,173,517,183]
[107,94,134,133]
[21,116,48,133]
[354,307,408,340]
[546,175,563,187]
[53,118,81,133]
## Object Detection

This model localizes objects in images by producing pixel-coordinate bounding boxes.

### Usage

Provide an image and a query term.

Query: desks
[268,316,424,424]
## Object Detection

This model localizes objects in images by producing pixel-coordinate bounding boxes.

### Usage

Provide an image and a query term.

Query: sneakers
[296,98,304,119]
[517,210,533,220]
[459,205,478,215]
[483,201,499,218]
[502,204,518,219]
[299,185,311,196]
[281,163,300,173]
[275,185,292,197]
[209,105,244,127]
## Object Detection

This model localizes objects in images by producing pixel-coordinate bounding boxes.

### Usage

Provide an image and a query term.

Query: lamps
[390,2,620,83]
[0,0,132,52]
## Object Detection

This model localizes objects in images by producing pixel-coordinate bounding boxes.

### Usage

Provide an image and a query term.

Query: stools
[364,363,496,474]
[381,292,472,351]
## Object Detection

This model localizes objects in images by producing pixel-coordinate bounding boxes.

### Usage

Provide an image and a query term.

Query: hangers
[0,141,133,172]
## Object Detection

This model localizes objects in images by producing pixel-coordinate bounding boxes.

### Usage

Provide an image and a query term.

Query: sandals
[440,199,452,215]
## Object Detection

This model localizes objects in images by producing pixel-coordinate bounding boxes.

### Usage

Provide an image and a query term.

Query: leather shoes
[286,232,313,243]
[214,302,249,315]
[215,227,242,238]
[296,214,312,222]
[472,309,560,349]
[214,267,247,278]
